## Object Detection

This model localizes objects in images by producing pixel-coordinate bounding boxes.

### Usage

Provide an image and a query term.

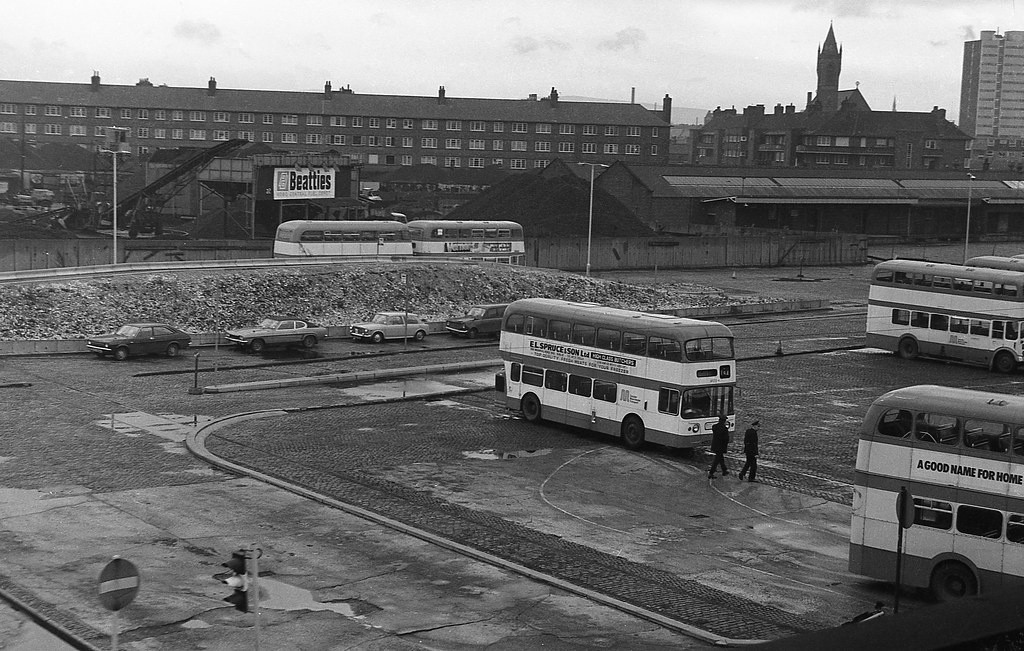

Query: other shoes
[708,473,714,478]
[748,479,758,482]
[722,471,729,476]
[739,473,743,480]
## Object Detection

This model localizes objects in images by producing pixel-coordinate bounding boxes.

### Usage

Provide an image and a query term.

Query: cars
[349,312,430,345]
[224,316,328,353]
[86,321,193,361]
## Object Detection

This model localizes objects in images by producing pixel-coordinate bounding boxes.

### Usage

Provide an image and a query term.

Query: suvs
[445,303,522,342]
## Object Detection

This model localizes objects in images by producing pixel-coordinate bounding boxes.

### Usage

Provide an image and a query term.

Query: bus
[848,385,1024,607]
[272,219,413,262]
[958,255,1024,298]
[404,220,526,264]
[494,296,743,455]
[863,259,1024,374]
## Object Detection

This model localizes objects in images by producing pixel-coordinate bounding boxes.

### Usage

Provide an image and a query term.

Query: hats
[751,421,759,426]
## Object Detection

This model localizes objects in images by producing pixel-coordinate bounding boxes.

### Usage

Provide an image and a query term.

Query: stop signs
[98,558,141,612]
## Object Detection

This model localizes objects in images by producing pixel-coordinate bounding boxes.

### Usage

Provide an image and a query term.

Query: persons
[707,414,729,479]
[739,420,761,482]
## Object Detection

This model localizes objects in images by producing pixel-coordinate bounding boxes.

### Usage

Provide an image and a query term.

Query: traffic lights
[221,550,249,615]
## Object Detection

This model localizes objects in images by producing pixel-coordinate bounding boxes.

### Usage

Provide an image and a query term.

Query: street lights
[964,171,977,264]
[99,149,133,265]
[577,161,610,277]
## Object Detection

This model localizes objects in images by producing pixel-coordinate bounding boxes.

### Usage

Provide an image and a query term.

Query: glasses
[755,425,760,427]
